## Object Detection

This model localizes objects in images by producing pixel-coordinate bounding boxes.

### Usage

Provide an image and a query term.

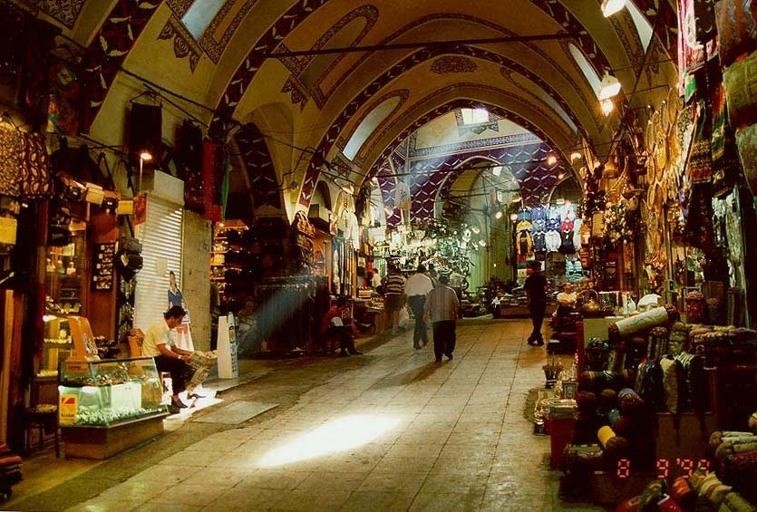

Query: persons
[379,265,405,336]
[404,265,434,350]
[318,296,364,359]
[369,262,437,290]
[551,282,579,317]
[166,270,191,350]
[511,260,550,347]
[421,275,463,366]
[140,305,208,409]
[448,266,463,320]
[489,288,510,311]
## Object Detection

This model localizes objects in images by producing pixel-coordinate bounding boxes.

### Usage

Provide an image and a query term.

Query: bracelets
[176,353,182,360]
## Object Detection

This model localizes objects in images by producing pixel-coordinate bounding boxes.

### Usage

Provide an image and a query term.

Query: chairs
[127,327,164,387]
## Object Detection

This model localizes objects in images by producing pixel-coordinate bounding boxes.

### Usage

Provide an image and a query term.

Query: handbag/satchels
[397,303,410,328]
[112,212,145,285]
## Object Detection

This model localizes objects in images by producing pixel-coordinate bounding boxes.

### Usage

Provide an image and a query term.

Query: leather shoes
[412,338,430,350]
[168,396,189,414]
[338,348,364,357]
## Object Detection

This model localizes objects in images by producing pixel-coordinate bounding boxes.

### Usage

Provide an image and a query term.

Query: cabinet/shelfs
[58,356,171,459]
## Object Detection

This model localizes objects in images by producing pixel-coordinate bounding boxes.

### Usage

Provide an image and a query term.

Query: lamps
[595,59,675,101]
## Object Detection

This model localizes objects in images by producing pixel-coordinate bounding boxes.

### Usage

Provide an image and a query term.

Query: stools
[21,404,61,461]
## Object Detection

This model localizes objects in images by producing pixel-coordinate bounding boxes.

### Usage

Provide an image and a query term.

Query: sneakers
[525,334,546,346]
[432,350,454,367]
[379,325,400,336]
